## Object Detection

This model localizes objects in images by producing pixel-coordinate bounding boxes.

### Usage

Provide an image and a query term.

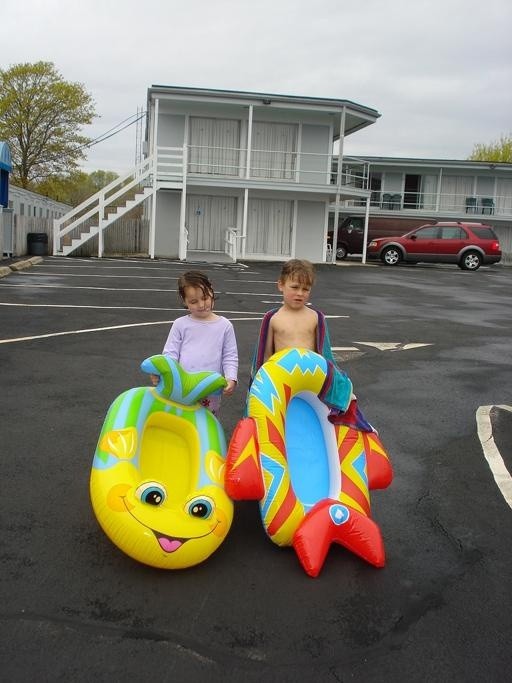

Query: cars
[367,222,502,270]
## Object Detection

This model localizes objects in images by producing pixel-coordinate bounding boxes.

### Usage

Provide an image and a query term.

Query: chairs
[465,197,479,214]
[481,198,495,216]
[381,193,402,211]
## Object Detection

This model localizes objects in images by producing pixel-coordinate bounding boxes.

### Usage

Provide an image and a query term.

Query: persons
[250,259,335,368]
[151,269,239,415]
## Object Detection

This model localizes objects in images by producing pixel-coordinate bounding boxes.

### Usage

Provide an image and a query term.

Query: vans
[327,215,436,261]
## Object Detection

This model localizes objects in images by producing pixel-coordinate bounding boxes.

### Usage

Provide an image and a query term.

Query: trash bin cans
[27,233,48,256]
[382,192,402,210]
